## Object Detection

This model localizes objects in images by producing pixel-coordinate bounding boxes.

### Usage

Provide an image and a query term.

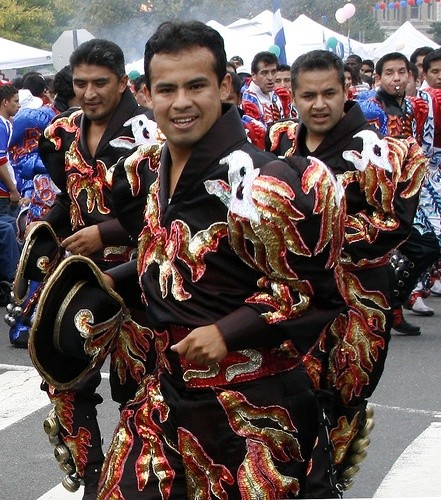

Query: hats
[28,254,124,391]
[18,89,43,110]
[13,220,61,305]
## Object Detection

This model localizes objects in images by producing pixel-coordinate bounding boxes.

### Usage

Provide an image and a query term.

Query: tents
[0,10,441,71]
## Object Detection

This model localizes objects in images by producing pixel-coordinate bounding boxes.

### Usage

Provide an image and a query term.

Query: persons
[10,39,167,500]
[264,50,430,499]
[26,21,350,500]
[0,46,441,348]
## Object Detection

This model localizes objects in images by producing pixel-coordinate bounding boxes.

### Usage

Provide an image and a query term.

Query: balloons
[267,45,280,58]
[327,36,338,49]
[335,0,441,24]
[128,70,140,79]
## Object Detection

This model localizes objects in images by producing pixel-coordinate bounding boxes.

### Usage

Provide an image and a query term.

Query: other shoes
[390,320,421,336]
[412,297,434,312]
